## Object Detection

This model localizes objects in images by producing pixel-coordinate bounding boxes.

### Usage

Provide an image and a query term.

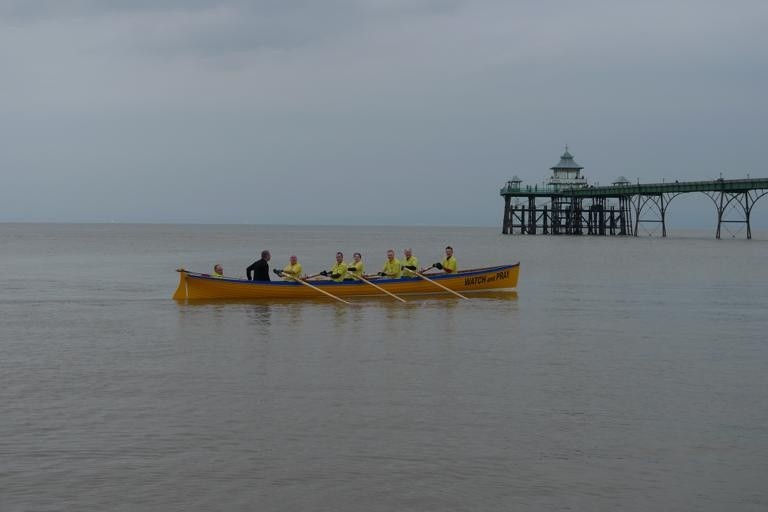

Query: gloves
[273,269,284,275]
[433,263,442,269]
[377,272,386,276]
[320,270,328,276]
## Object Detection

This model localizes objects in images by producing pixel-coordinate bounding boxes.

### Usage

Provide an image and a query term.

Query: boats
[172,263,520,302]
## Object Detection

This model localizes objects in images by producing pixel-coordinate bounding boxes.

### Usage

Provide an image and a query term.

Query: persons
[440,246,458,274]
[281,255,303,281]
[246,250,272,282]
[378,249,401,279]
[347,252,364,281]
[209,264,225,279]
[325,251,347,282]
[401,248,420,279]
